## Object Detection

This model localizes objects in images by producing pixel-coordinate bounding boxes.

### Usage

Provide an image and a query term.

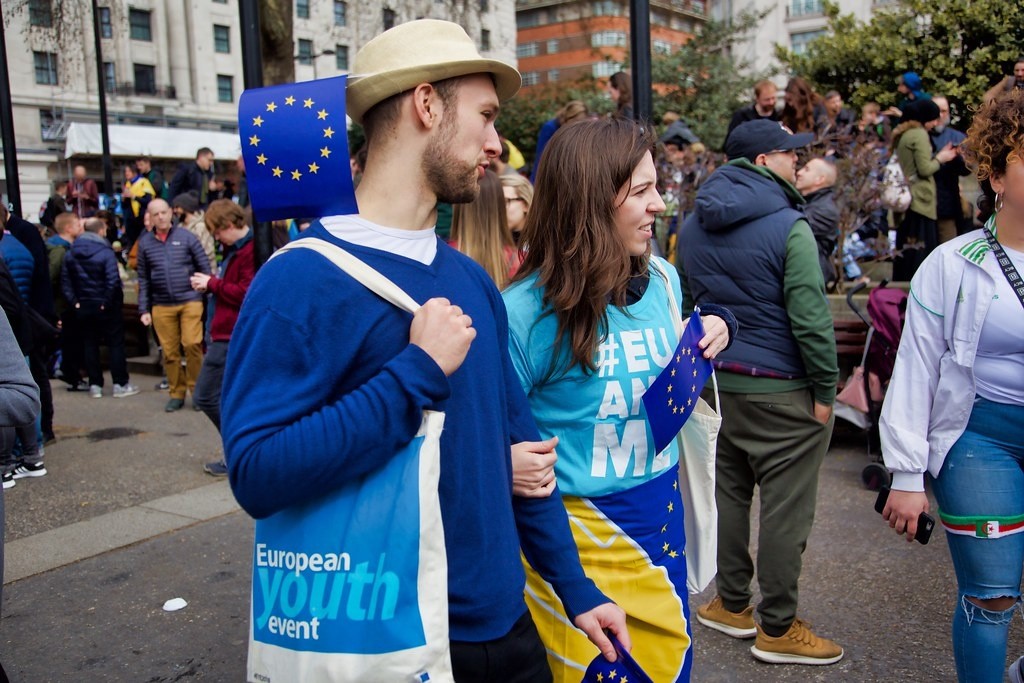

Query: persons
[529,102,586,184]
[237,193,290,275]
[0,302,42,683]
[609,71,632,112]
[675,119,846,665]
[0,147,255,489]
[496,115,739,683]
[491,135,529,172]
[651,56,1024,294]
[447,165,537,295]
[219,17,631,683]
[877,85,1024,683]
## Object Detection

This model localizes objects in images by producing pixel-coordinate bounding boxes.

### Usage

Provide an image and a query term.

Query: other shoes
[204,461,228,476]
[167,396,186,412]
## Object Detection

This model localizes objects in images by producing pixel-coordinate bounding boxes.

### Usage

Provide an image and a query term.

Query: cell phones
[874,486,935,545]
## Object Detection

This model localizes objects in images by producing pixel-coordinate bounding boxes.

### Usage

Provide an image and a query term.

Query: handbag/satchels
[646,256,721,596]
[245,237,454,683]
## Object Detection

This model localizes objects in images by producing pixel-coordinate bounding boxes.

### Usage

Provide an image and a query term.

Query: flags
[642,310,716,455]
[238,72,361,223]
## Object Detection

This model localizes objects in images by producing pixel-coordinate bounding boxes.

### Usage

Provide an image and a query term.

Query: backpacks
[879,149,920,212]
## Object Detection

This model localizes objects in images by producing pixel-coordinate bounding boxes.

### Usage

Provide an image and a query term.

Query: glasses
[754,147,795,163]
[505,197,523,208]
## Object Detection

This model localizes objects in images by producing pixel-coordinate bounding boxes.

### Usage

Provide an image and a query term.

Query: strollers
[844,278,913,492]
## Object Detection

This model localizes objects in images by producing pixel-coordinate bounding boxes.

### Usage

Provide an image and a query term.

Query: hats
[727,120,814,158]
[345,18,523,125]
[171,190,199,211]
[901,99,939,123]
[903,73,920,89]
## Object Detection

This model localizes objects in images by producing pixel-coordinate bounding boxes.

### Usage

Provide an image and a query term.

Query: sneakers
[113,383,141,397]
[750,617,844,663]
[11,460,47,478]
[89,383,101,397]
[2,472,16,489]
[697,593,759,637]
[156,379,170,391]
